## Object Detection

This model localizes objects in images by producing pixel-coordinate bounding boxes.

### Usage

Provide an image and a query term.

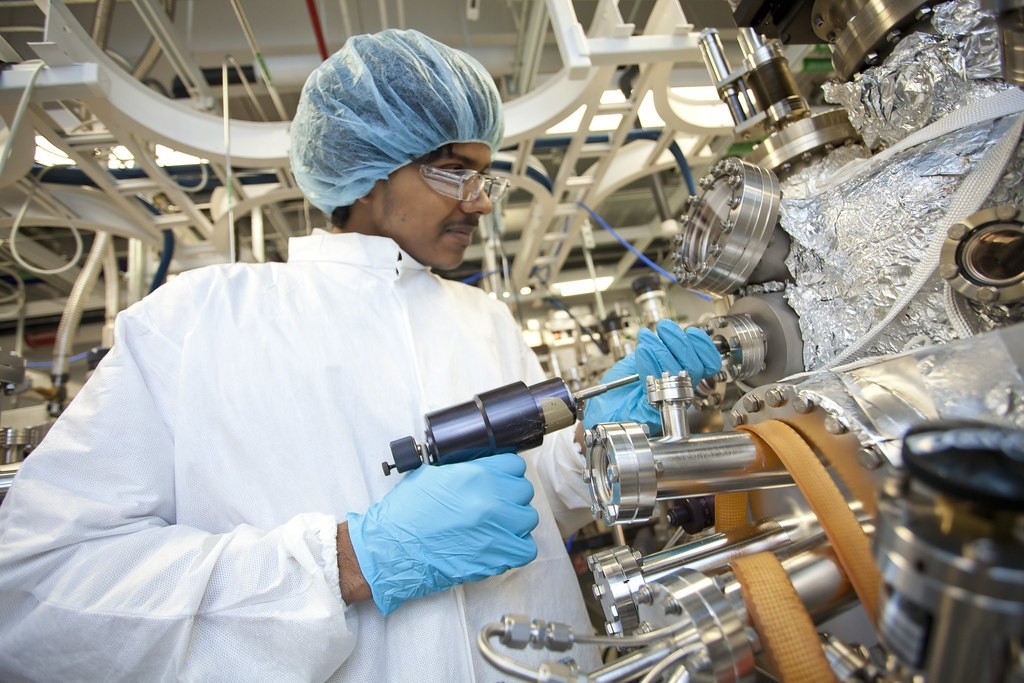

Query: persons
[1,28,723,680]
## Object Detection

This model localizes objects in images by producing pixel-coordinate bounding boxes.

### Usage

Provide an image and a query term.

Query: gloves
[579,319,722,440]
[347,454,539,619]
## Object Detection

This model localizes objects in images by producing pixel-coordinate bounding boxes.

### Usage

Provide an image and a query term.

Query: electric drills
[380,372,641,476]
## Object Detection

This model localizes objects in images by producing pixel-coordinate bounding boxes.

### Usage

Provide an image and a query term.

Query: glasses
[407,156,510,202]
